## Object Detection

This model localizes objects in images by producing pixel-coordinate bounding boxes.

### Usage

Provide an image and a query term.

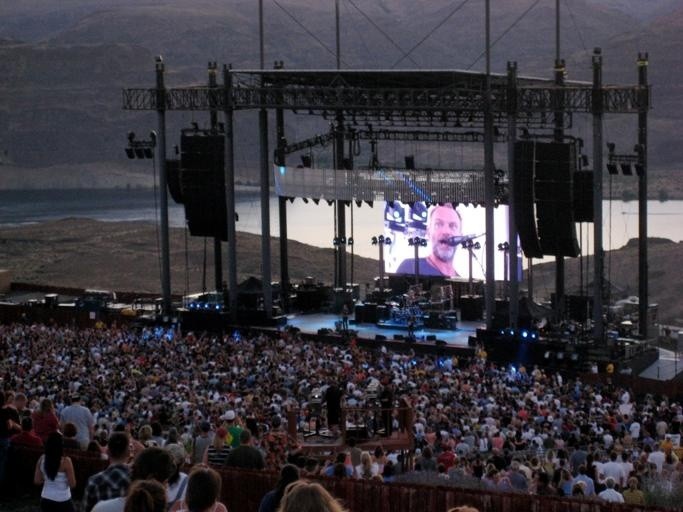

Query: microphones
[448,232,484,246]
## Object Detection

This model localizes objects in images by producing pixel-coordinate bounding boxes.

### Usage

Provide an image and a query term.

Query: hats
[223,410,235,420]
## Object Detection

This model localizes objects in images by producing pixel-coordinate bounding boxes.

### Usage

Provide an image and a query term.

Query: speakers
[394,335,403,340]
[181,135,203,236]
[199,134,228,237]
[165,159,184,203]
[436,340,447,345]
[376,335,386,340]
[427,336,435,340]
[513,140,543,258]
[535,141,580,258]
[318,330,329,334]
[291,328,300,331]
[572,170,594,222]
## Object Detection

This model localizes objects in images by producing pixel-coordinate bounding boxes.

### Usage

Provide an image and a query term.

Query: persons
[397,204,465,279]
